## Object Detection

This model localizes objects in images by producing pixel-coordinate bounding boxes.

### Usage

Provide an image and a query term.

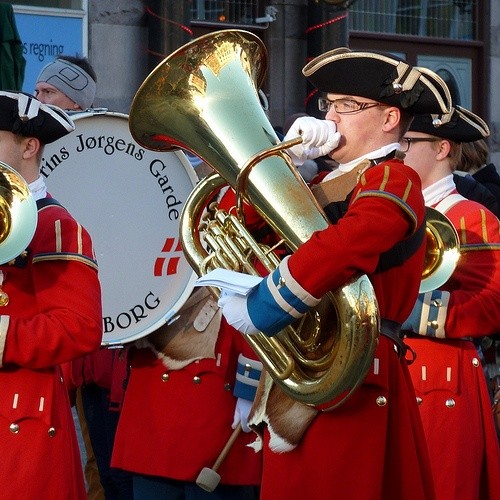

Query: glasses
[398,137,452,158]
[317,97,390,113]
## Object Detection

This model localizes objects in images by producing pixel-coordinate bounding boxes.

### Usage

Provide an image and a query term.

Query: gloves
[231,397,255,433]
[217,289,261,334]
[281,116,341,166]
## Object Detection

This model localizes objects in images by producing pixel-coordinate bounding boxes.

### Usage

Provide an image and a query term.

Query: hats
[408,104,490,145]
[301,47,452,114]
[0,90,76,144]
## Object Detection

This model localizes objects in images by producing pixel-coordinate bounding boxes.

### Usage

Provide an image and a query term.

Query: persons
[393,106,500,500]
[0,0,340,500]
[453,141,500,439]
[216,47,452,500]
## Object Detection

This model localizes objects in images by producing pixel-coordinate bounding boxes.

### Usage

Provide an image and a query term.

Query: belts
[462,336,472,341]
[376,318,400,337]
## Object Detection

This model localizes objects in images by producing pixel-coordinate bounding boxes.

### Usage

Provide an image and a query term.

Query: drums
[37,110,201,347]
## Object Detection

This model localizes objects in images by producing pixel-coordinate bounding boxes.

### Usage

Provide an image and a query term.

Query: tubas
[129,29,382,413]
[418,206,500,292]
[0,160,38,265]
